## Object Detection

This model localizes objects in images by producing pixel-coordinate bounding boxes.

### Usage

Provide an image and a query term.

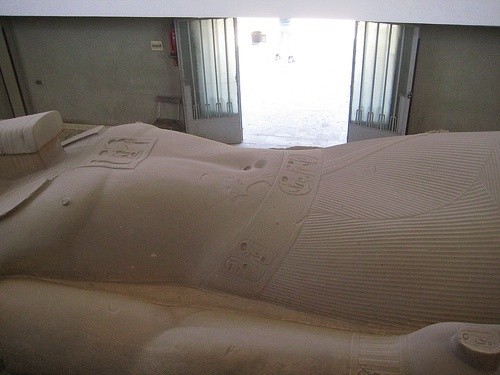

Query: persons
[0,109,500,374]
